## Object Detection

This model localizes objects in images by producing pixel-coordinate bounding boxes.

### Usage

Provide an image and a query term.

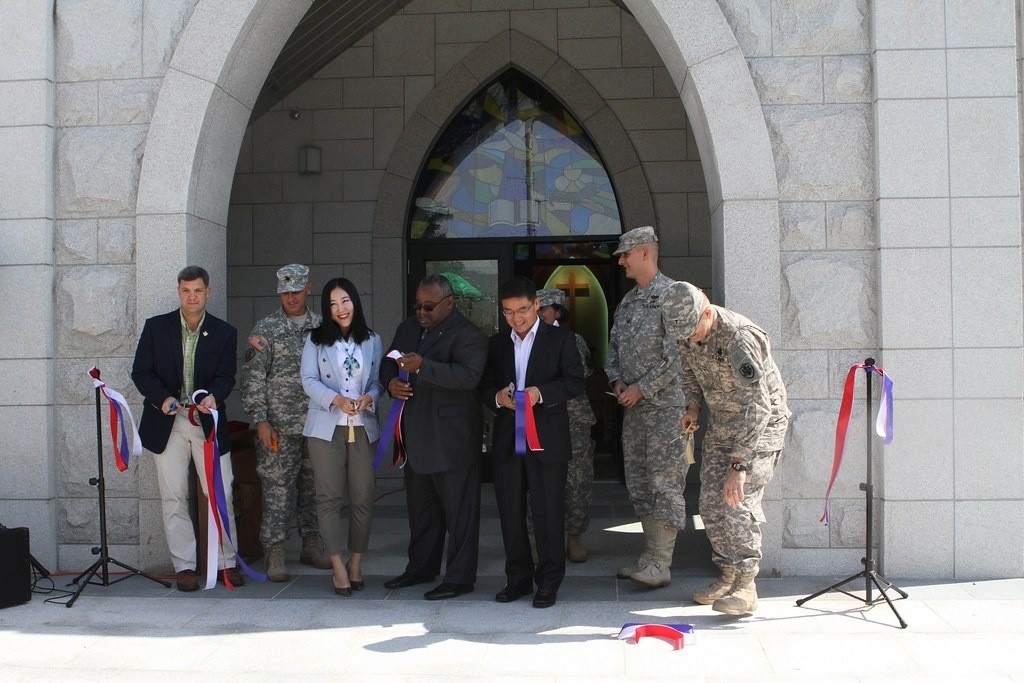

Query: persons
[301,277,386,597]
[132,266,243,592]
[241,263,334,581]
[478,277,584,608]
[381,274,490,600]
[527,288,595,565]
[659,281,792,616]
[604,225,702,588]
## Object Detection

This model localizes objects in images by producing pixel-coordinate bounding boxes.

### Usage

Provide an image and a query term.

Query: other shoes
[345,560,363,590]
[335,586,352,597]
[568,534,587,562]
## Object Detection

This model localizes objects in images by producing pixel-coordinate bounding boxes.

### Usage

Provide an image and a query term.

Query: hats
[612,226,658,256]
[276,263,310,294]
[658,280,704,340]
[536,288,566,307]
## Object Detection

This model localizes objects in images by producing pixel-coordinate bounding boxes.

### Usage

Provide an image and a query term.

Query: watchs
[732,461,746,471]
[415,368,420,375]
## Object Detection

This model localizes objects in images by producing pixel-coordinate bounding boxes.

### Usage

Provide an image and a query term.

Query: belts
[179,404,192,408]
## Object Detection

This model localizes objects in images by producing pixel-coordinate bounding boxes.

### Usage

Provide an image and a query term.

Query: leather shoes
[424,582,471,601]
[496,578,535,602]
[385,572,435,589]
[534,585,560,608]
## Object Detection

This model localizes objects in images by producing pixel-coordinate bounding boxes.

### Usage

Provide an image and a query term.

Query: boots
[630,520,678,587]
[617,514,658,578]
[300,537,333,569]
[268,542,289,582]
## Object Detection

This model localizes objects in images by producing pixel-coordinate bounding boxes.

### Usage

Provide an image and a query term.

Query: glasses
[503,302,533,318]
[413,295,450,311]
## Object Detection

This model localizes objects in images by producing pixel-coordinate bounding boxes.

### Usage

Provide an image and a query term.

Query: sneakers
[712,575,758,615]
[177,569,199,591]
[693,567,735,604]
[219,569,243,586]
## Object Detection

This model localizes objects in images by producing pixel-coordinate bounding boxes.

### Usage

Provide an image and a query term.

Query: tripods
[65,366,173,608]
[796,357,909,627]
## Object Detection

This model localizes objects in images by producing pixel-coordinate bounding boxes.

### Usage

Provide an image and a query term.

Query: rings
[732,490,738,492]
[400,362,404,368]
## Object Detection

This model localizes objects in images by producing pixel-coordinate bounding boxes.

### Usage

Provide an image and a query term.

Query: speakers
[0,526,32,609]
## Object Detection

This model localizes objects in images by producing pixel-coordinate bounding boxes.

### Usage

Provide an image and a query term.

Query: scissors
[606,392,618,398]
[507,383,516,402]
[669,424,692,443]
[165,400,178,416]
